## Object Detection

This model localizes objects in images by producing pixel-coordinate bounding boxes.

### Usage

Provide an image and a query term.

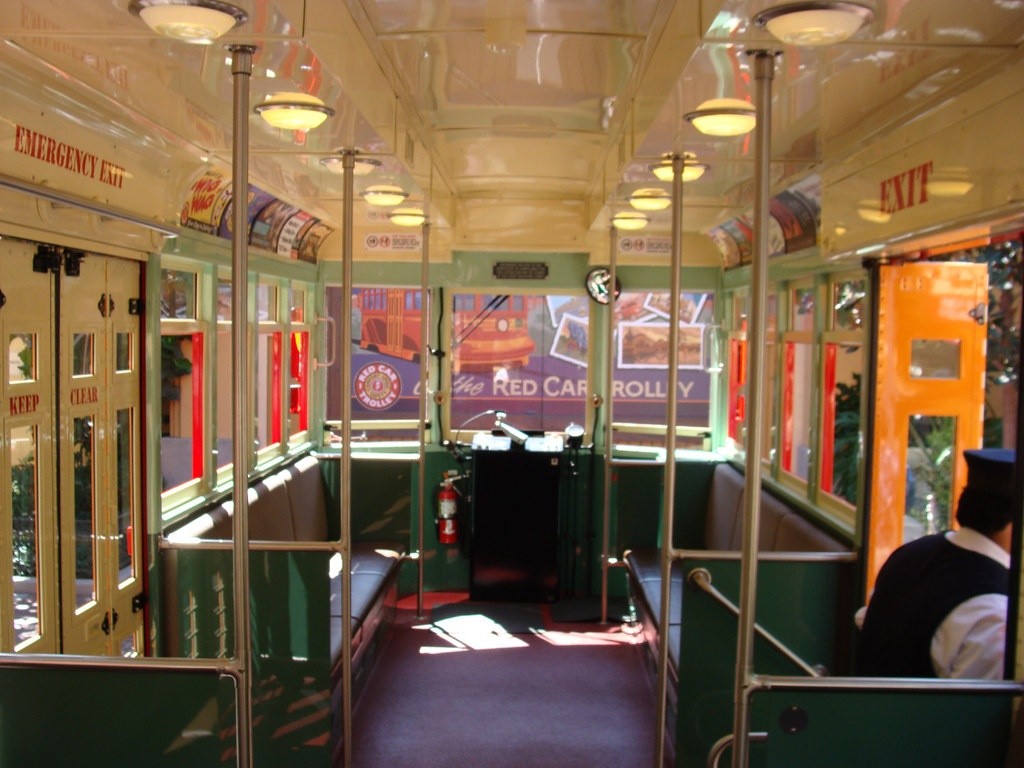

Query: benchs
[613,464,850,719]
[160,450,404,709]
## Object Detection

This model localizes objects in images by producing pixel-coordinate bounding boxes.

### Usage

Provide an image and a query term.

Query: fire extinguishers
[436,476,464,545]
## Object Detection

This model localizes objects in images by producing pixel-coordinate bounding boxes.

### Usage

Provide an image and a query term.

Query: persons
[845,451,1016,681]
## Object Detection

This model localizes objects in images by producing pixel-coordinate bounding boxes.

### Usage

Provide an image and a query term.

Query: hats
[963,449,1016,495]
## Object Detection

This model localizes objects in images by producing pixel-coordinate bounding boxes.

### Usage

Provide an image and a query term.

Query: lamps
[127,0,248,44]
[358,184,410,206]
[386,208,428,226]
[751,0,875,47]
[648,152,710,182]
[319,146,381,176]
[625,188,672,211]
[252,92,336,129]
[610,212,651,230]
[682,97,757,137]
